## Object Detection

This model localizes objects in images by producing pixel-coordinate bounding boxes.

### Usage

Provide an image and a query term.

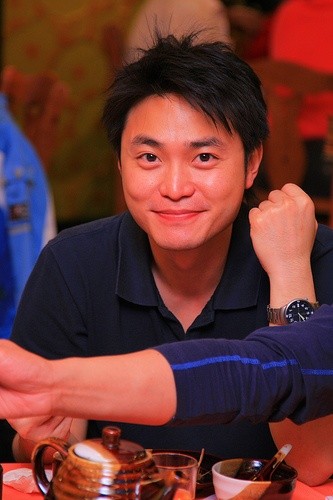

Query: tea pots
[31,427,186,500]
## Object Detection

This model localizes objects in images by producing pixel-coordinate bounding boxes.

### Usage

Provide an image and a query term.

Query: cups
[139,452,198,500]
[212,459,298,500]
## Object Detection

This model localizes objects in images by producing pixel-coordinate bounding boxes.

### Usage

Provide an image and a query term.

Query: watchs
[265,297,316,325]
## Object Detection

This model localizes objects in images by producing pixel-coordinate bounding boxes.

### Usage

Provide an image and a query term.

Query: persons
[0,16,333,489]
[0,0,333,500]
